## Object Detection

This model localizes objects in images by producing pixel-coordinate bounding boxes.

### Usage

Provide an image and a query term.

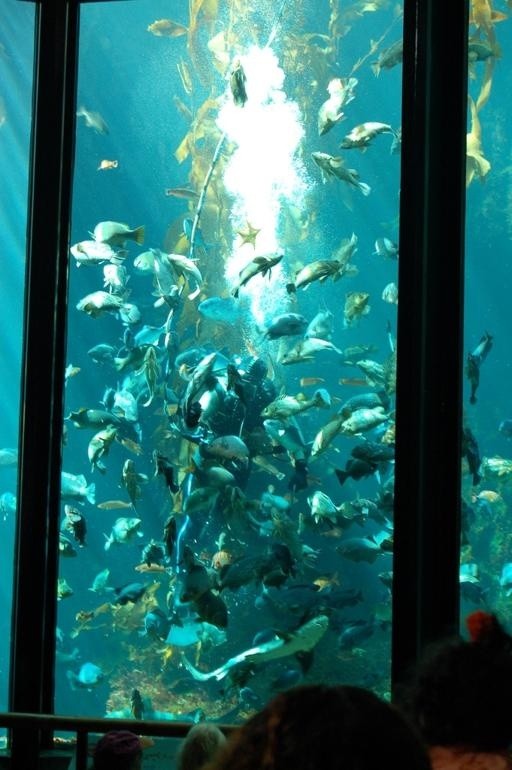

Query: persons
[411,630,512,768]
[92,731,141,766]
[206,684,432,768]
[176,724,226,768]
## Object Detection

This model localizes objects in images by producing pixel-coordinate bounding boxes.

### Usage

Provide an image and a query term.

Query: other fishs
[55,0,404,725]
